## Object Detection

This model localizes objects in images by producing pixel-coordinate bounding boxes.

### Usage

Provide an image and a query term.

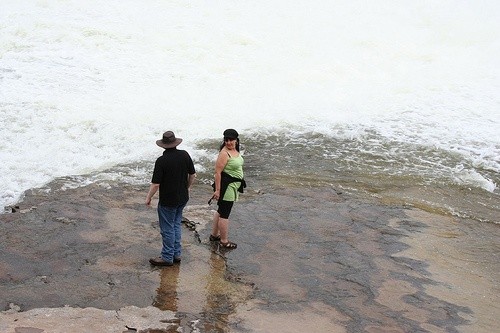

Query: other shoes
[149,256,173,266]
[174,256,181,262]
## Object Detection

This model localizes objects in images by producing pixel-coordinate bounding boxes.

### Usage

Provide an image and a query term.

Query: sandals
[219,240,237,248]
[209,234,220,241]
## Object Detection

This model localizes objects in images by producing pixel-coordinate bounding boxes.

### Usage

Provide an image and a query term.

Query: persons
[145,131,196,265]
[208,129,247,248]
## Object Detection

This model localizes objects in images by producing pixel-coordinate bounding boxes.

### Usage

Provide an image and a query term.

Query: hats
[224,128,238,139]
[156,131,182,149]
